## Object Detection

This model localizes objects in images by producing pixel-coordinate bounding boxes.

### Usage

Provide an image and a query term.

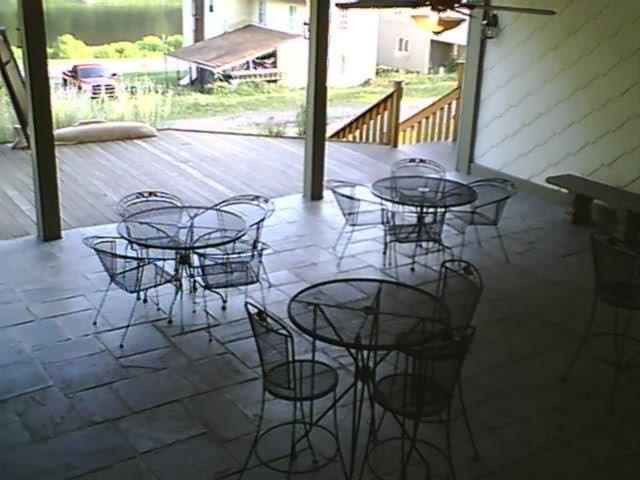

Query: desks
[286,277,452,477]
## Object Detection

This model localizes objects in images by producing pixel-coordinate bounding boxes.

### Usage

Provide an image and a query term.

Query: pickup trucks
[61,63,122,100]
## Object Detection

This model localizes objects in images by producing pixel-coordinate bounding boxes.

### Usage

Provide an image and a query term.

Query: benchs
[543,170,638,241]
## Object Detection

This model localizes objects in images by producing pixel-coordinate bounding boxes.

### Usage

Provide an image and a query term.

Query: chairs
[320,152,519,275]
[395,257,484,394]
[557,231,640,413]
[238,300,349,477]
[359,323,483,478]
[80,188,278,351]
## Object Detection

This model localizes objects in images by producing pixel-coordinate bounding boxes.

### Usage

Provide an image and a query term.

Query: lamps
[480,12,498,40]
[411,15,467,35]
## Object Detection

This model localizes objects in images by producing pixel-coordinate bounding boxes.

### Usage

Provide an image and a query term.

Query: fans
[332,0,557,20]
